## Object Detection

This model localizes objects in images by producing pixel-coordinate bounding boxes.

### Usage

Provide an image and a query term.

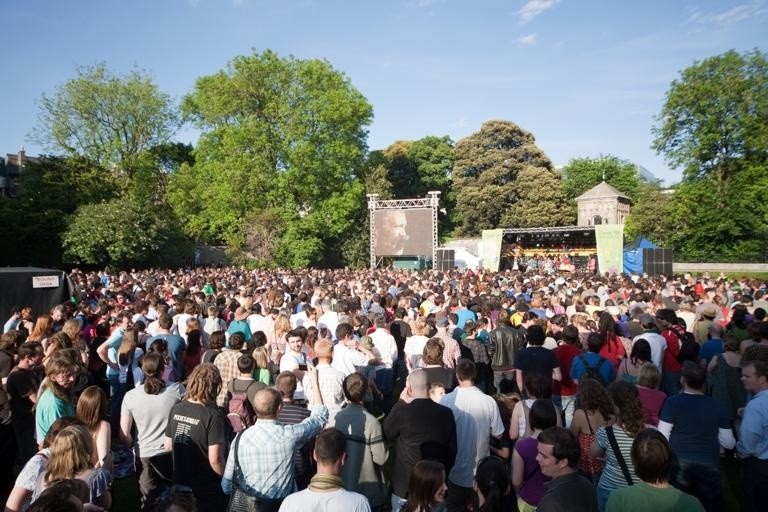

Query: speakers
[642,247,673,275]
[437,249,455,271]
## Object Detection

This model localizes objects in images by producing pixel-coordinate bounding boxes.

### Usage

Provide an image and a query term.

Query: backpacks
[225,379,255,434]
[668,326,696,365]
[579,355,604,384]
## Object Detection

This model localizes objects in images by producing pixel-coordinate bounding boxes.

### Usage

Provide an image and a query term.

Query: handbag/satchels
[226,473,281,512]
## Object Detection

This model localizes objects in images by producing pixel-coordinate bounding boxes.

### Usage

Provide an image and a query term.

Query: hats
[234,306,250,322]
[360,335,375,348]
[702,305,719,318]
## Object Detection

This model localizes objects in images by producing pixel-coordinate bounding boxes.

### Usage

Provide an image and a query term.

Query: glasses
[477,455,503,466]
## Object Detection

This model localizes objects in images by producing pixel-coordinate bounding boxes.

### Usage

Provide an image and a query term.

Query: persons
[0,255,768,512]
[375,209,409,255]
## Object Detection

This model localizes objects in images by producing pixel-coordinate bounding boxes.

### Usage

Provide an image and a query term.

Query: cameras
[299,364,307,371]
